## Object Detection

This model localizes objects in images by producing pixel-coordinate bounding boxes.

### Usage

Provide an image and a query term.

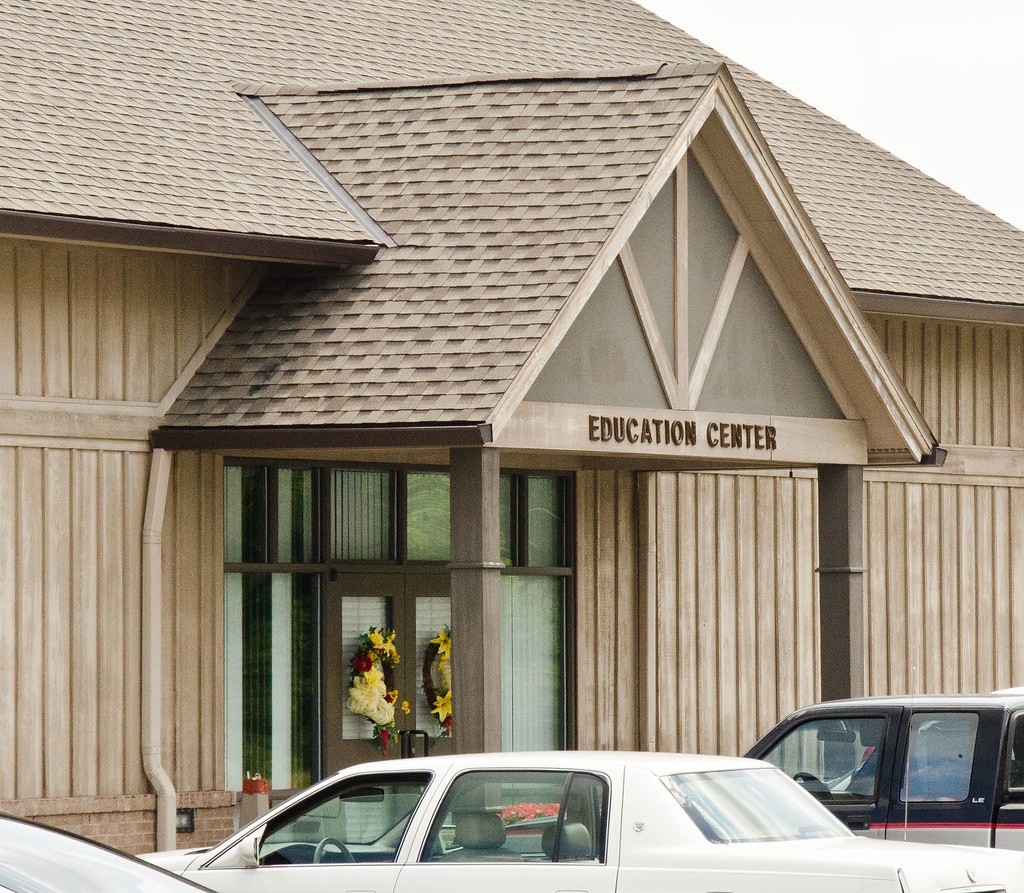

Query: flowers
[347,623,452,755]
[496,802,563,825]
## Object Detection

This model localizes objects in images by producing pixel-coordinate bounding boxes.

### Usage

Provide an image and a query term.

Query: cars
[133,750,1024,893]
[741,693,1024,852]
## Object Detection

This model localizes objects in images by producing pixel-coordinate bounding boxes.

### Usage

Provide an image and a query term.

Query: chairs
[438,813,599,863]
